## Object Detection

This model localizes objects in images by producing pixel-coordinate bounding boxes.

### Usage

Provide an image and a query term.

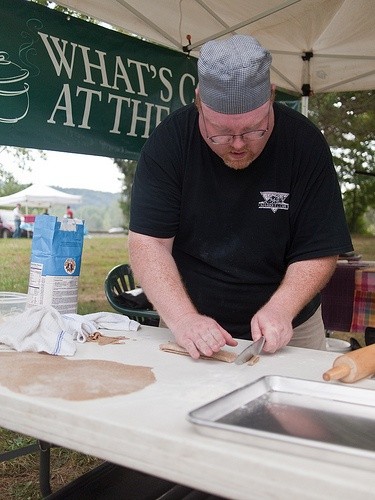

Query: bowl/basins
[0,291,27,315]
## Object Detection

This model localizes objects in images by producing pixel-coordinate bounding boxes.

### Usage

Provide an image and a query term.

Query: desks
[321,261,375,333]
[0,326,375,500]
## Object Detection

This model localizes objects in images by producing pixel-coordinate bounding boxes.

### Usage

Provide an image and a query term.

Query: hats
[198,34,271,114]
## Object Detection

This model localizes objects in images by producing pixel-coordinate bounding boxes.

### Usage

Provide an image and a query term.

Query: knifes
[233,335,266,365]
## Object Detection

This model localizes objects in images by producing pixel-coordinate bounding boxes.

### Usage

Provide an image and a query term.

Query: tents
[0,183,83,238]
[0,0,375,161]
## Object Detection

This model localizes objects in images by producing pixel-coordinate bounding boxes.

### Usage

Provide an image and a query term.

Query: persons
[126,33,354,359]
[66,206,73,219]
[42,208,49,215]
[12,204,24,238]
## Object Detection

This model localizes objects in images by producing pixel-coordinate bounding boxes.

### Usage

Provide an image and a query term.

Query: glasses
[201,96,271,145]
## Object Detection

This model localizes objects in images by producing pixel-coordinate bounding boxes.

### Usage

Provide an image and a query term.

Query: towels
[0,303,142,356]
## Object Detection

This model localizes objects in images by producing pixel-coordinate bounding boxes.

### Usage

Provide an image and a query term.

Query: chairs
[104,263,161,327]
